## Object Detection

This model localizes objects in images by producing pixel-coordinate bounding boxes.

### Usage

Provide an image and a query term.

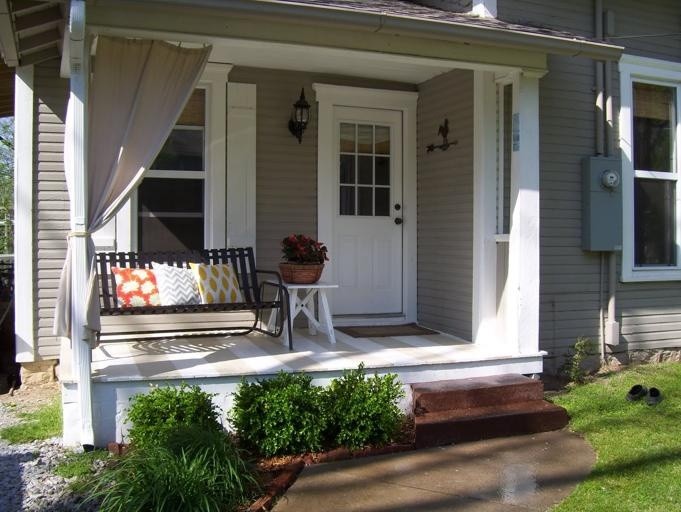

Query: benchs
[90,247,293,362]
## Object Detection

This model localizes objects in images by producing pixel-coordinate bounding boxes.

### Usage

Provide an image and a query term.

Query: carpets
[333,322,440,338]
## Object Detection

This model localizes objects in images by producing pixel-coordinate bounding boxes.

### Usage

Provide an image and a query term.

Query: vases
[279,260,325,284]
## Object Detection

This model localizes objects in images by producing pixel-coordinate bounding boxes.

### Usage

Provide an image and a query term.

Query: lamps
[288,88,311,144]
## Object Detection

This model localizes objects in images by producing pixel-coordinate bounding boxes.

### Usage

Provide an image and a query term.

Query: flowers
[283,234,329,265]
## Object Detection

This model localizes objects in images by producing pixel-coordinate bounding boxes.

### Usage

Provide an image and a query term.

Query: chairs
[111,261,242,308]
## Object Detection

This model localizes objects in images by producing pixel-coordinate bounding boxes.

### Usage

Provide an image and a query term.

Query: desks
[266,280,339,345]
